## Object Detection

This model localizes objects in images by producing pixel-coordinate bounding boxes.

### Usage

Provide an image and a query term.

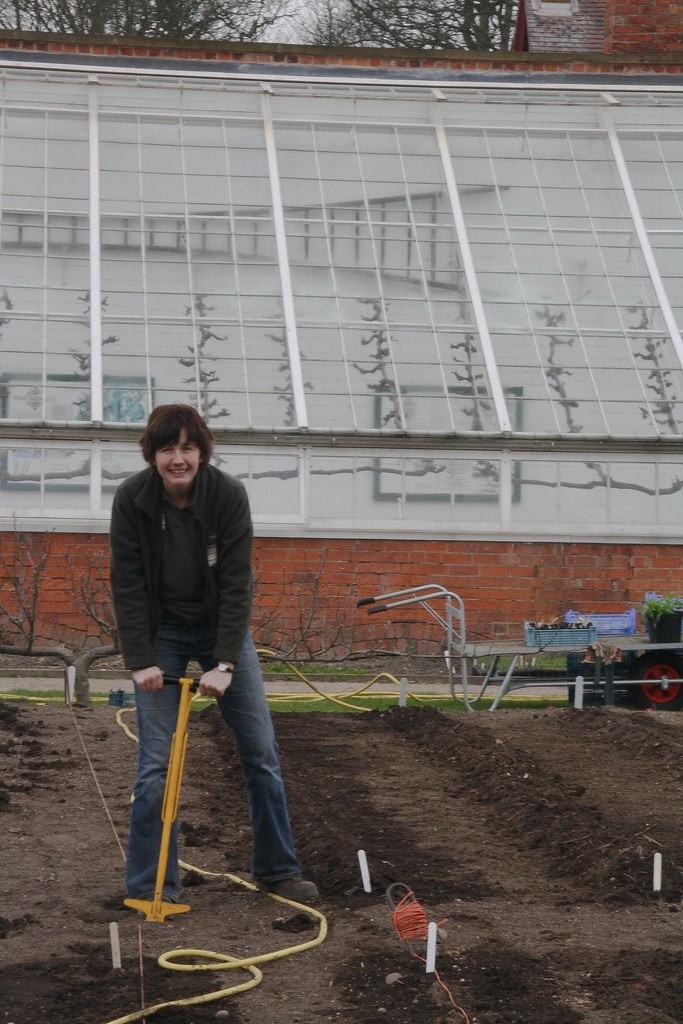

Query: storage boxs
[525,622,597,647]
[644,592,683,635]
[567,650,638,707]
[566,609,636,636]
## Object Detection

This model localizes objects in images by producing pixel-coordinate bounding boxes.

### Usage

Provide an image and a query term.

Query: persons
[109,404,319,919]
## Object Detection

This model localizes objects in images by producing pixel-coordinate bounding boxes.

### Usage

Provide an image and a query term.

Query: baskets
[565,609,635,633]
[524,621,596,646]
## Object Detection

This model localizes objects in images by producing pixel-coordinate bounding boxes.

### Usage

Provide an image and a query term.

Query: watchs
[218,663,233,673]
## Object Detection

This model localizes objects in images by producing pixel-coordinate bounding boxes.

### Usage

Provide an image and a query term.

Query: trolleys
[353,582,683,714]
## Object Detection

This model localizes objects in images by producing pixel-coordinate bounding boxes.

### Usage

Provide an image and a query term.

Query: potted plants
[638,591,683,643]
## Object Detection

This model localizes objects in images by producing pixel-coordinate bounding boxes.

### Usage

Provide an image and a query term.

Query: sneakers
[256,877,319,900]
[136,896,175,922]
